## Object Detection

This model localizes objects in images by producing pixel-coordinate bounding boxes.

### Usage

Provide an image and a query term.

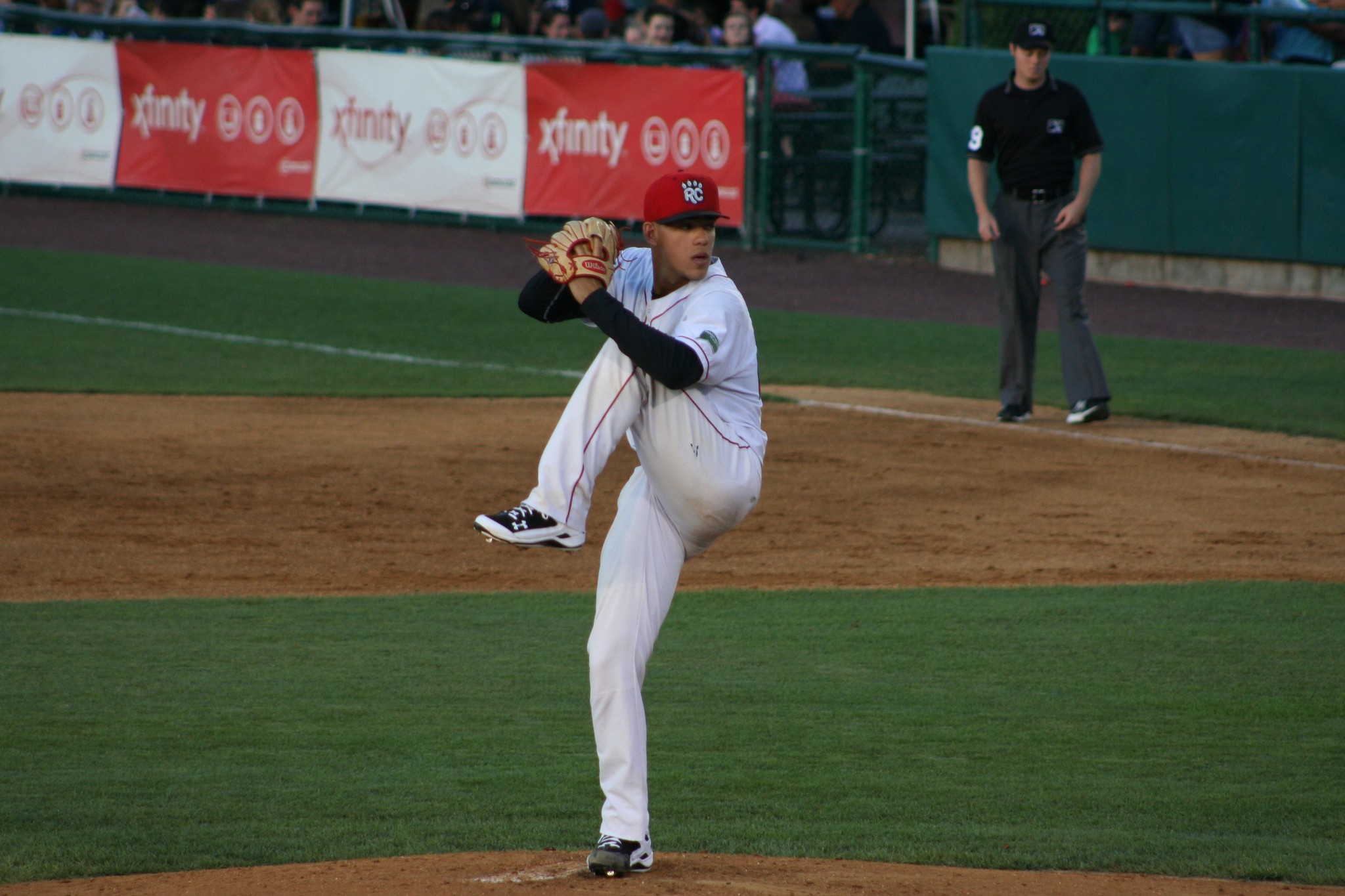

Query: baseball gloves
[533,217,623,288]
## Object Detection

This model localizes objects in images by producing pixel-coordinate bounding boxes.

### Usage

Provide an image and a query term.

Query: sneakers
[997,404,1034,422]
[474,504,585,550]
[1067,398,1112,424]
[587,834,654,875]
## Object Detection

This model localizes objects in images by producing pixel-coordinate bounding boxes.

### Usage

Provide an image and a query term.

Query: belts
[1002,186,1072,201]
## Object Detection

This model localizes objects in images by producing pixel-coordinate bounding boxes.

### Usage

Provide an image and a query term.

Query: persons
[472,167,768,882]
[965,22,1113,427]
[1,0,1345,152]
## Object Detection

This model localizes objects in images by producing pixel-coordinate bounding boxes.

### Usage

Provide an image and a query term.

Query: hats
[642,169,730,222]
[1008,19,1053,51]
